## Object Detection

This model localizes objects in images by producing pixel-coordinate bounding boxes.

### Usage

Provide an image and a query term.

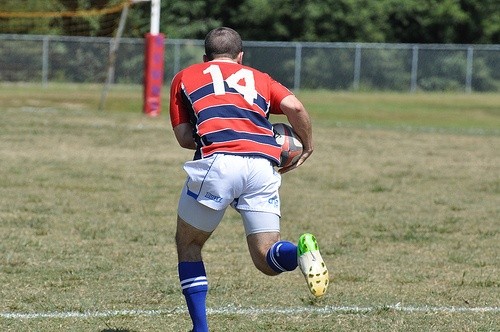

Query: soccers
[272,122,303,169]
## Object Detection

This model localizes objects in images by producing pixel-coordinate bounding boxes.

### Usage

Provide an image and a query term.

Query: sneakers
[296,233,329,296]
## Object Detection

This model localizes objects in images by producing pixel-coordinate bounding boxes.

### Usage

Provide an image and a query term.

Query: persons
[167,26,329,332]
